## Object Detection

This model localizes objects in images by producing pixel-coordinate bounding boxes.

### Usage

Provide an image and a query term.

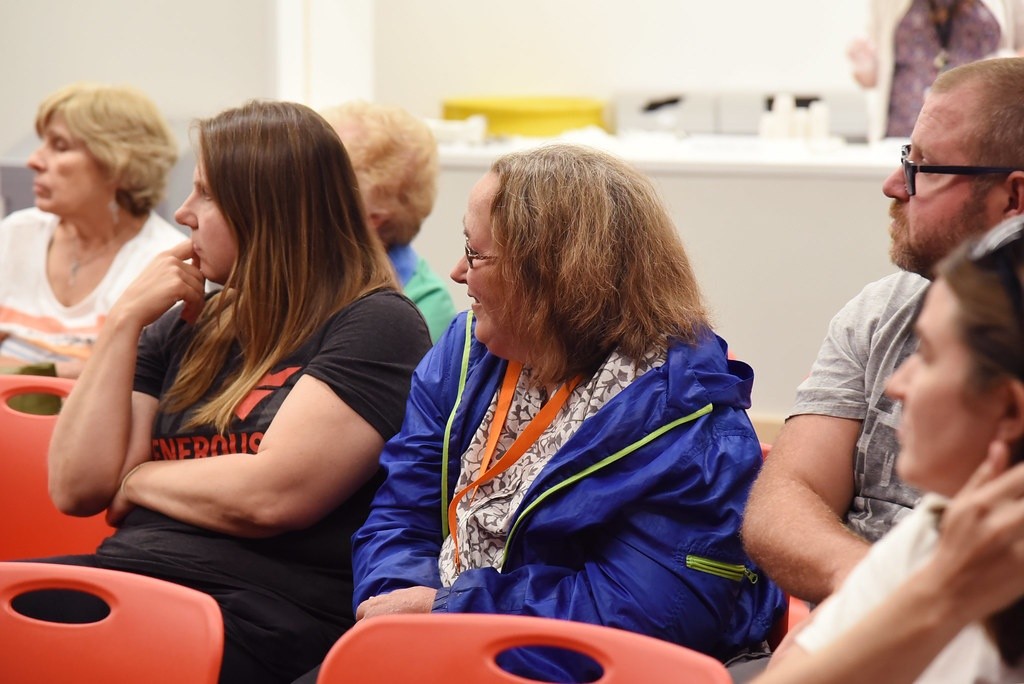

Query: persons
[887,0,1002,138]
[0,53,1024,684]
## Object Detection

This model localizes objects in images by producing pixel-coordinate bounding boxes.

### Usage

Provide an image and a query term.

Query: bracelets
[121,461,147,501]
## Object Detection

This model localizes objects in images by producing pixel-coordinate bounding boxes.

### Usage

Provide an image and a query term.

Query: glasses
[465,236,522,268]
[901,144,1024,196]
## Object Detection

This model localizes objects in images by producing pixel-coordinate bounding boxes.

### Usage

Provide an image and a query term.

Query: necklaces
[51,214,130,287]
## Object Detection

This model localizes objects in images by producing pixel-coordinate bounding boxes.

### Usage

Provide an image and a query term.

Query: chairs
[0,373,117,561]
[317,613,731,684]
[0,562,224,684]
